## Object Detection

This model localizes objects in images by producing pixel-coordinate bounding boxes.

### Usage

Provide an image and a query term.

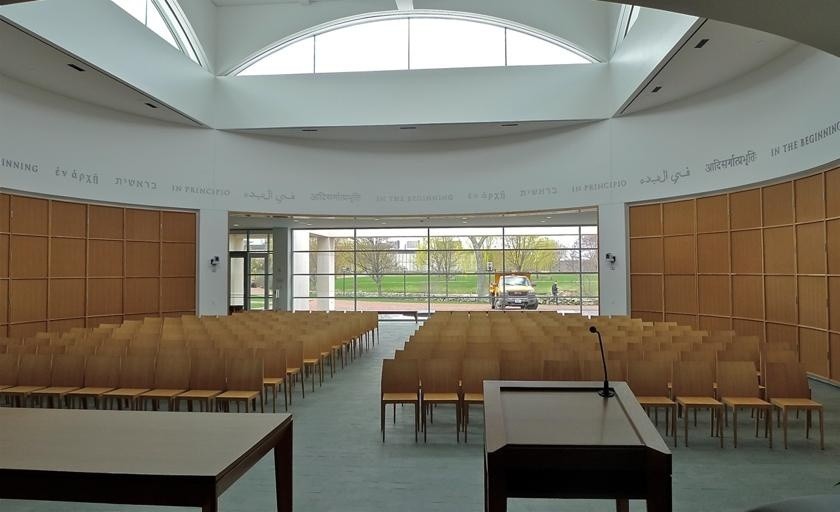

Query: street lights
[402,266,409,295]
[341,266,348,296]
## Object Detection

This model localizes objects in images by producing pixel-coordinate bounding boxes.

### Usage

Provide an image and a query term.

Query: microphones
[589,326,615,398]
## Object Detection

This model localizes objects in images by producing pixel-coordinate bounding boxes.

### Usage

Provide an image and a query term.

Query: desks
[1,406,296,511]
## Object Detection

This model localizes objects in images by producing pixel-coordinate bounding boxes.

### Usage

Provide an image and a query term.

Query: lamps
[604,252,616,264]
[209,254,221,266]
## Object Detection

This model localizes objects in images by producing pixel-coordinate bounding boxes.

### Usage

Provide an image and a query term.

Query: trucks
[488,270,541,311]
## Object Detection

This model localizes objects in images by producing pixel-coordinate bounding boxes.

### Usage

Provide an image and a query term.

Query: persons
[548,281,558,305]
